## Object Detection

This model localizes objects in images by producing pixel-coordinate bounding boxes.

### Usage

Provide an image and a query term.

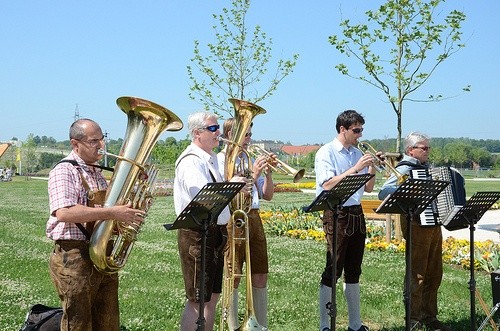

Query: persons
[378,131,443,331]
[174,111,249,331]
[46,118,145,331]
[315,110,386,331]
[217,118,278,331]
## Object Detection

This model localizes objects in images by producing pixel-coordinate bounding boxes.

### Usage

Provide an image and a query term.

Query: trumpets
[247,143,305,182]
[358,139,410,185]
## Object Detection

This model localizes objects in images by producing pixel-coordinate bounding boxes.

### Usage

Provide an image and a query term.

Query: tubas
[225,209,271,331]
[87,96,184,272]
[215,97,256,216]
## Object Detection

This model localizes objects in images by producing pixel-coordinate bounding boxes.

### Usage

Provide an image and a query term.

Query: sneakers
[348,325,369,331]
[322,327,332,331]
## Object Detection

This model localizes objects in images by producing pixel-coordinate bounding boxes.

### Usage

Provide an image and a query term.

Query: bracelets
[353,167,359,174]
[264,171,272,176]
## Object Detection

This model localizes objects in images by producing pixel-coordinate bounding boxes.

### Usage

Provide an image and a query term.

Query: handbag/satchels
[19,304,63,331]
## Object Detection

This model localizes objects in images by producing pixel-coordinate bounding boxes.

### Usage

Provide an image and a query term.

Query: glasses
[413,146,431,150]
[245,132,252,137]
[75,135,105,144]
[197,125,220,132]
[349,128,364,134]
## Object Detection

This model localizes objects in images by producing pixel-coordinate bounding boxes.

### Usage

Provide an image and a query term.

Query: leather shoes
[429,320,451,331]
